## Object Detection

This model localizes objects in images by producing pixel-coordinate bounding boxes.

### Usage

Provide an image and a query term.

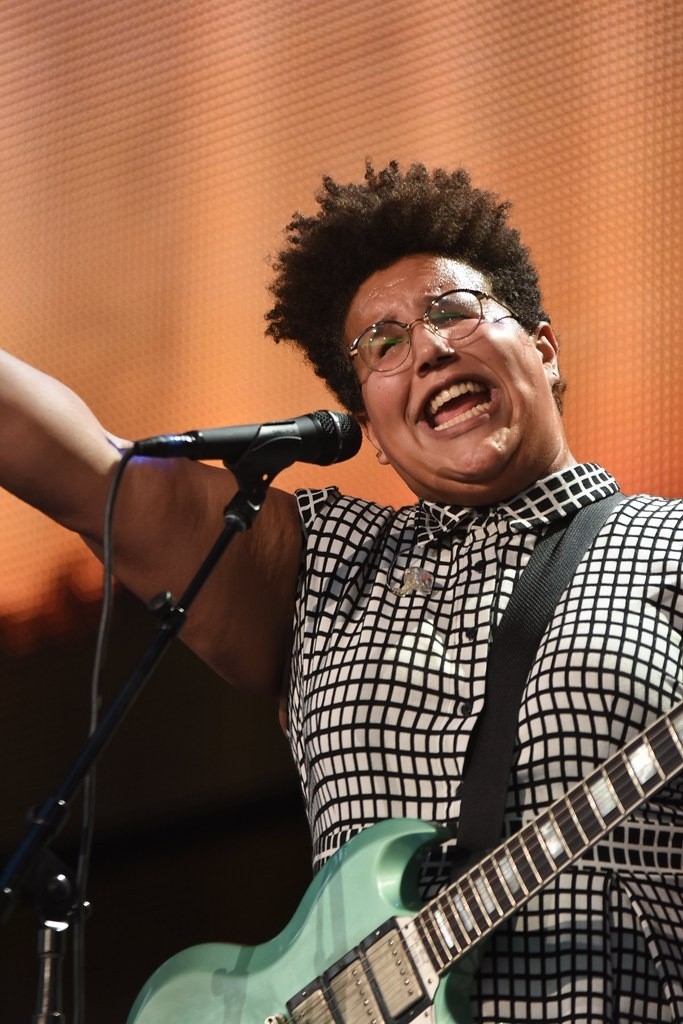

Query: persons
[0,157,683,1023]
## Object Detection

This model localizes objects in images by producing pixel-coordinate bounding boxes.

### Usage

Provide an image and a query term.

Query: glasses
[343,286,523,399]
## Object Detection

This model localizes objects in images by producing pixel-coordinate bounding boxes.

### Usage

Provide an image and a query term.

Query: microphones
[132,409,363,466]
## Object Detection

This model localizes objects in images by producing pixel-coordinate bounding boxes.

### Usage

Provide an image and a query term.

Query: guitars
[126,700,682,1024]
